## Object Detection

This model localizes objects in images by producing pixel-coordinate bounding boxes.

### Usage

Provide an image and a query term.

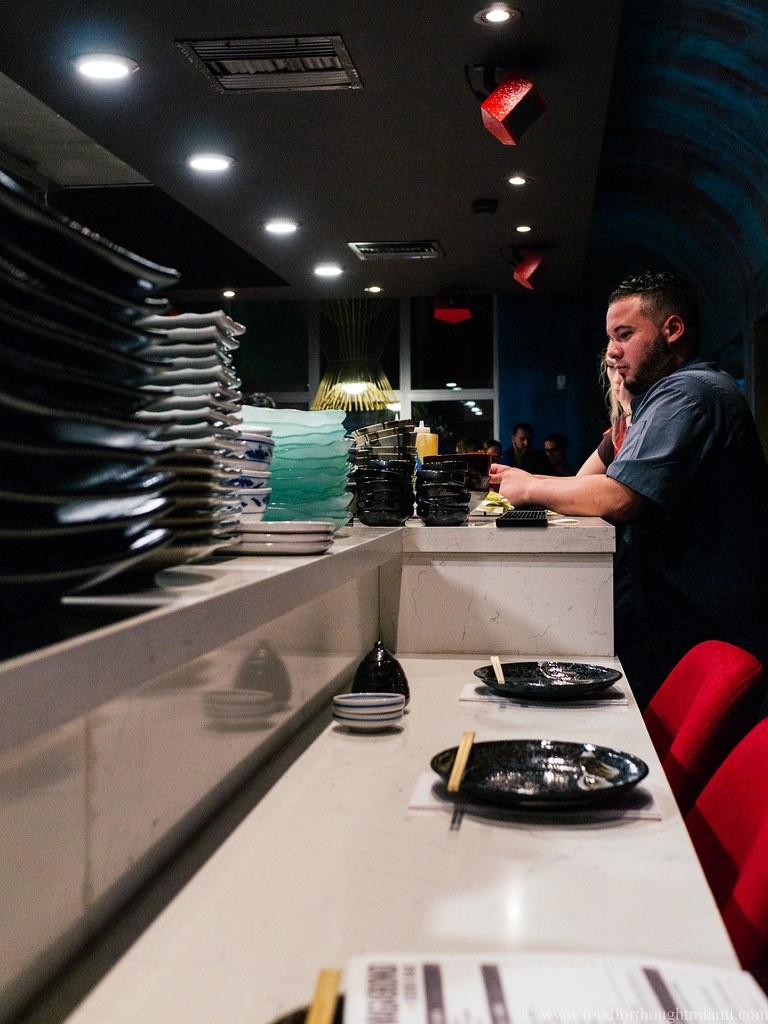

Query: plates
[430,739,648,810]
[204,690,276,730]
[473,661,623,701]
[227,404,354,535]
[0,170,179,628]
[135,309,246,577]
[215,521,336,555]
[332,693,405,732]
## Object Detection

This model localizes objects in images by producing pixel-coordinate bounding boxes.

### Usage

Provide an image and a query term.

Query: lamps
[462,55,548,146]
[500,245,550,289]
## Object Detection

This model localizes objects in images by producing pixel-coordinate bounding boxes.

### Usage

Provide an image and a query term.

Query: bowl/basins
[223,425,276,521]
[346,419,418,527]
[415,454,493,527]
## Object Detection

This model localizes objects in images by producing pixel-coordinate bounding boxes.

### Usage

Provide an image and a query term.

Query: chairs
[639,640,768,812]
[683,717,768,972]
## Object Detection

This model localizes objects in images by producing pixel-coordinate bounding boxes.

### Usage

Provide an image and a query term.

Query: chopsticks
[490,655,505,684]
[306,969,340,1024]
[447,731,475,791]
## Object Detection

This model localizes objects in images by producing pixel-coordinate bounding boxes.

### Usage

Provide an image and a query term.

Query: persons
[487,265,768,716]
[456,422,578,477]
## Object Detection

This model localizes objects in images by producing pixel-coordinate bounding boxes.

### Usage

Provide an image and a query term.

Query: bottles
[415,420,438,465]
[238,639,292,708]
[351,641,410,708]
